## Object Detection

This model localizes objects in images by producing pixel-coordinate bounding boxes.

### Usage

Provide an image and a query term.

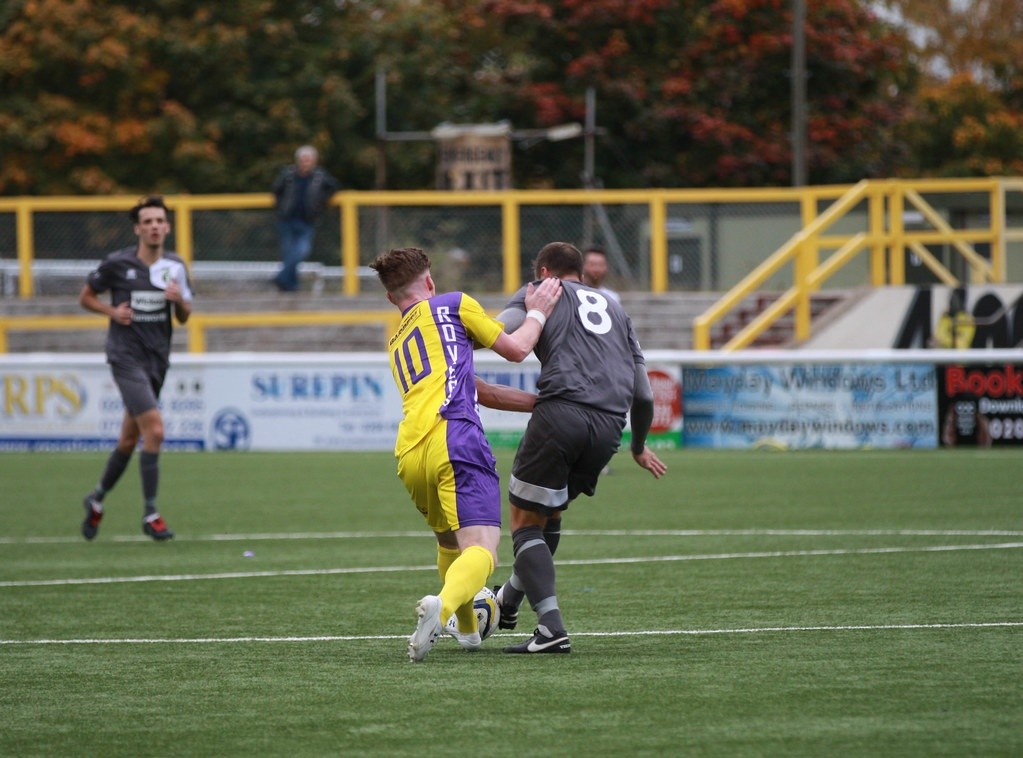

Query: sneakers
[502,627,571,654]
[491,586,518,630]
[142,512,174,541]
[444,613,482,651]
[81,495,104,539]
[407,595,444,661]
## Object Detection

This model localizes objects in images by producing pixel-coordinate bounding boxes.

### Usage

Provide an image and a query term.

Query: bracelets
[526,310,547,329]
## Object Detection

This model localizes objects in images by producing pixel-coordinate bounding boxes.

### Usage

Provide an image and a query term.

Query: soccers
[444,587,501,642]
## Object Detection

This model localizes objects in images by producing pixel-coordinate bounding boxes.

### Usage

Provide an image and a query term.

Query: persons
[80,197,194,539]
[369,246,562,663]
[471,243,666,653]
[583,247,621,476]
[271,145,343,291]
[936,290,977,349]
